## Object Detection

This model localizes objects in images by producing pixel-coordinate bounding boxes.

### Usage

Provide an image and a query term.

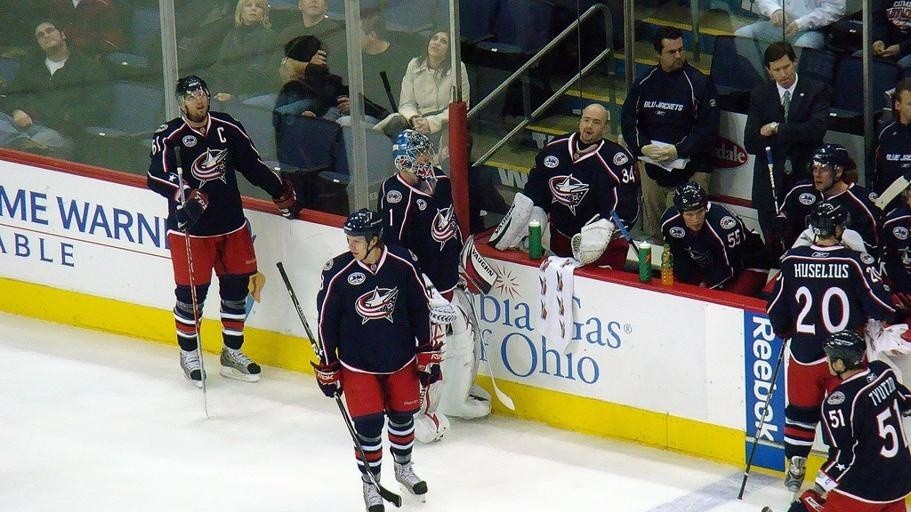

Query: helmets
[674,182,706,210]
[877,325,911,359]
[813,201,849,240]
[823,331,870,372]
[813,143,850,180]
[175,76,211,99]
[344,209,384,238]
[393,128,435,178]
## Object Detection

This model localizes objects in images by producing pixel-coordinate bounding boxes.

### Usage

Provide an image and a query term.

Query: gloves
[416,344,443,387]
[272,182,301,220]
[455,266,479,296]
[790,490,826,512]
[311,356,342,398]
[176,188,209,231]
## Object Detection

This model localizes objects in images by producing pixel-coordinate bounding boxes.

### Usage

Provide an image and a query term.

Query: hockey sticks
[173,145,216,421]
[735,335,789,502]
[467,289,517,411]
[275,261,403,508]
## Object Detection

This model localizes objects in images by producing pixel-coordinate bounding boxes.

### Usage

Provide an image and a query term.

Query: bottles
[639,240,651,283]
[528,219,541,260]
[661,244,674,286]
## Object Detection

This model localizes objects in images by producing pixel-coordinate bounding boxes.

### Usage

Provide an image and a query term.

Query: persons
[272,35,390,215]
[522,105,641,270]
[318,209,443,512]
[876,79,911,203]
[765,202,906,494]
[737,0,847,49]
[0,20,111,162]
[775,143,890,278]
[620,25,716,246]
[875,170,911,310]
[377,129,492,443]
[744,41,832,270]
[660,182,767,294]
[373,31,470,151]
[211,0,278,102]
[147,75,302,388]
[323,8,403,126]
[241,0,345,115]
[852,0,911,68]
[48,0,124,66]
[438,120,506,232]
[762,331,911,511]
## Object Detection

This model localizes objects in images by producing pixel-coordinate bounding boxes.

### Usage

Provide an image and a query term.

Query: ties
[783,90,790,122]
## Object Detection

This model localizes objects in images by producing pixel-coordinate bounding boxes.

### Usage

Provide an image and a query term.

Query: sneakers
[220,344,262,374]
[179,349,207,381]
[393,461,427,495]
[785,456,806,491]
[363,480,384,511]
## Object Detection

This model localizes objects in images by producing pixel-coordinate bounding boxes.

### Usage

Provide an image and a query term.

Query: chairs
[709,35,774,100]
[321,126,397,186]
[270,112,339,174]
[477,0,556,56]
[81,82,165,140]
[426,0,494,45]
[108,7,159,70]
[793,46,837,97]
[0,56,18,84]
[220,103,281,172]
[825,57,900,118]
[362,1,444,36]
